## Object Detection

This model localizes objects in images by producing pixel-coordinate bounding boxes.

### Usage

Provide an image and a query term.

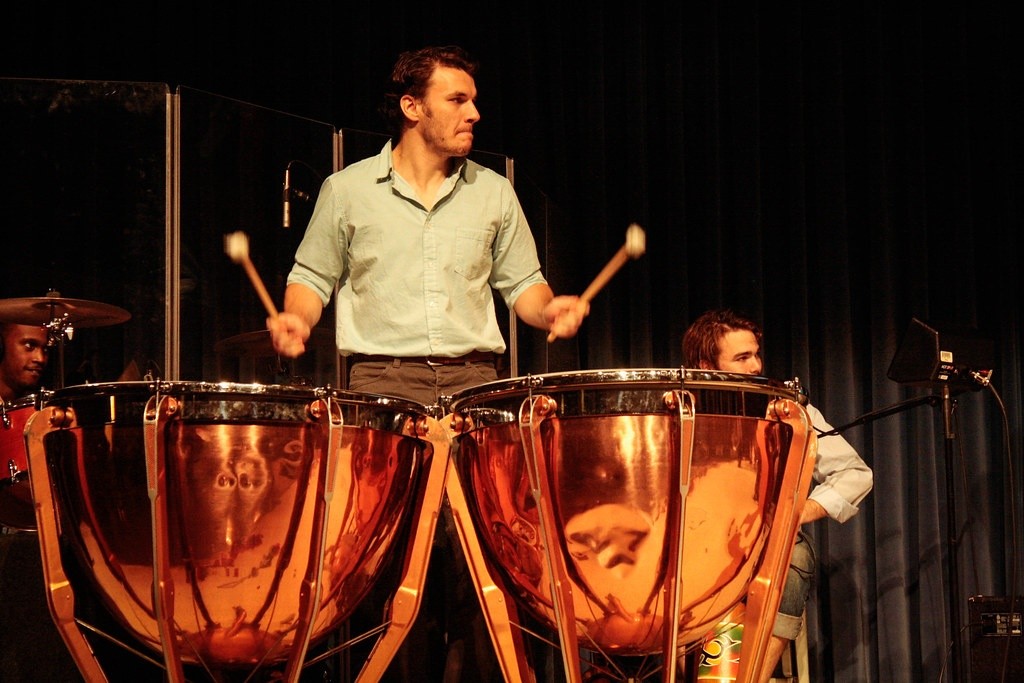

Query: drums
[0,385,56,534]
[20,377,452,682]
[433,364,818,682]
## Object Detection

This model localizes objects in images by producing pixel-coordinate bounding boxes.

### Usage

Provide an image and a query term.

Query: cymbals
[0,297,132,329]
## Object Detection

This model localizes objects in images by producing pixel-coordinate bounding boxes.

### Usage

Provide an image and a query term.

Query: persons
[0,321,50,479]
[677,316,875,683]
[265,47,590,415]
